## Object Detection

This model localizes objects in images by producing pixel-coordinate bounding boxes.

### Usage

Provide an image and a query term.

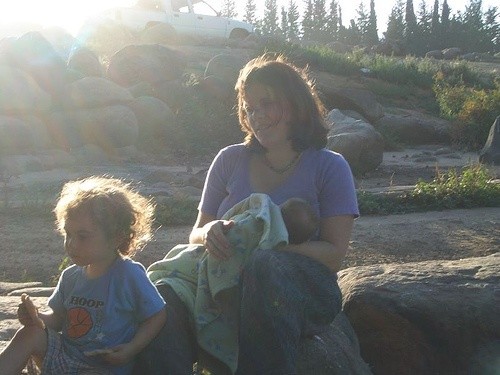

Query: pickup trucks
[109,0,254,40]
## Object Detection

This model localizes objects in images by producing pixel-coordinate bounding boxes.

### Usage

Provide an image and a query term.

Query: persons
[133,52,359,375]
[146,192,319,287]
[0,176,167,375]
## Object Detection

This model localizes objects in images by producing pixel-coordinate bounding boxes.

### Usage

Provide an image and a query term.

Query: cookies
[82,348,113,356]
[21,293,45,329]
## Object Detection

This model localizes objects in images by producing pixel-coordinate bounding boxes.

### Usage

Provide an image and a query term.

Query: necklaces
[259,151,302,175]
